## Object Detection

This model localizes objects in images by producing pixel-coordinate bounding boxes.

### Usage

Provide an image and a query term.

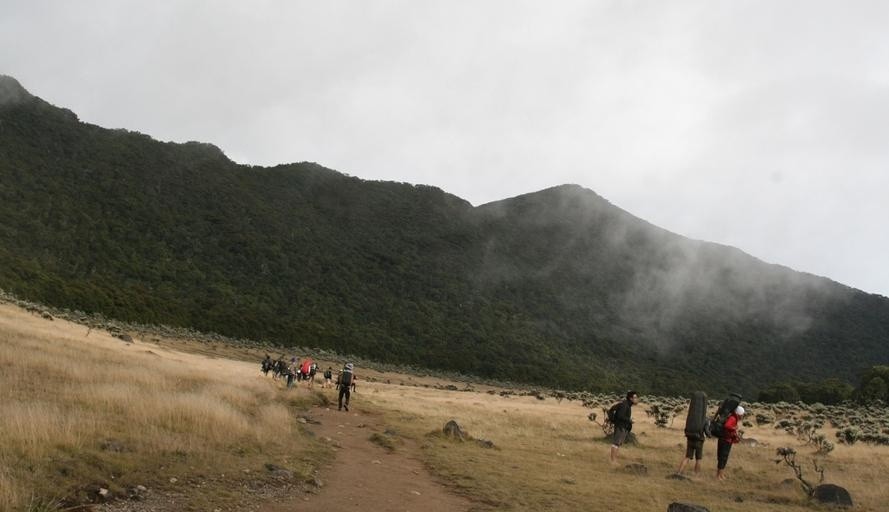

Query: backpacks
[343,370,351,386]
[607,402,630,423]
[685,390,708,439]
[710,392,743,438]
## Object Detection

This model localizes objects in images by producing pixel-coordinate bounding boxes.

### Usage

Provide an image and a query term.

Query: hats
[346,363,353,370]
[734,405,744,416]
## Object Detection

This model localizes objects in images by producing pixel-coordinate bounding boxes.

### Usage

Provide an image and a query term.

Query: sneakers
[344,404,348,411]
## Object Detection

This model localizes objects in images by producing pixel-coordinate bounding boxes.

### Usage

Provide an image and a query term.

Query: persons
[714,406,746,481]
[676,391,713,476]
[608,391,638,466]
[264,351,358,411]
